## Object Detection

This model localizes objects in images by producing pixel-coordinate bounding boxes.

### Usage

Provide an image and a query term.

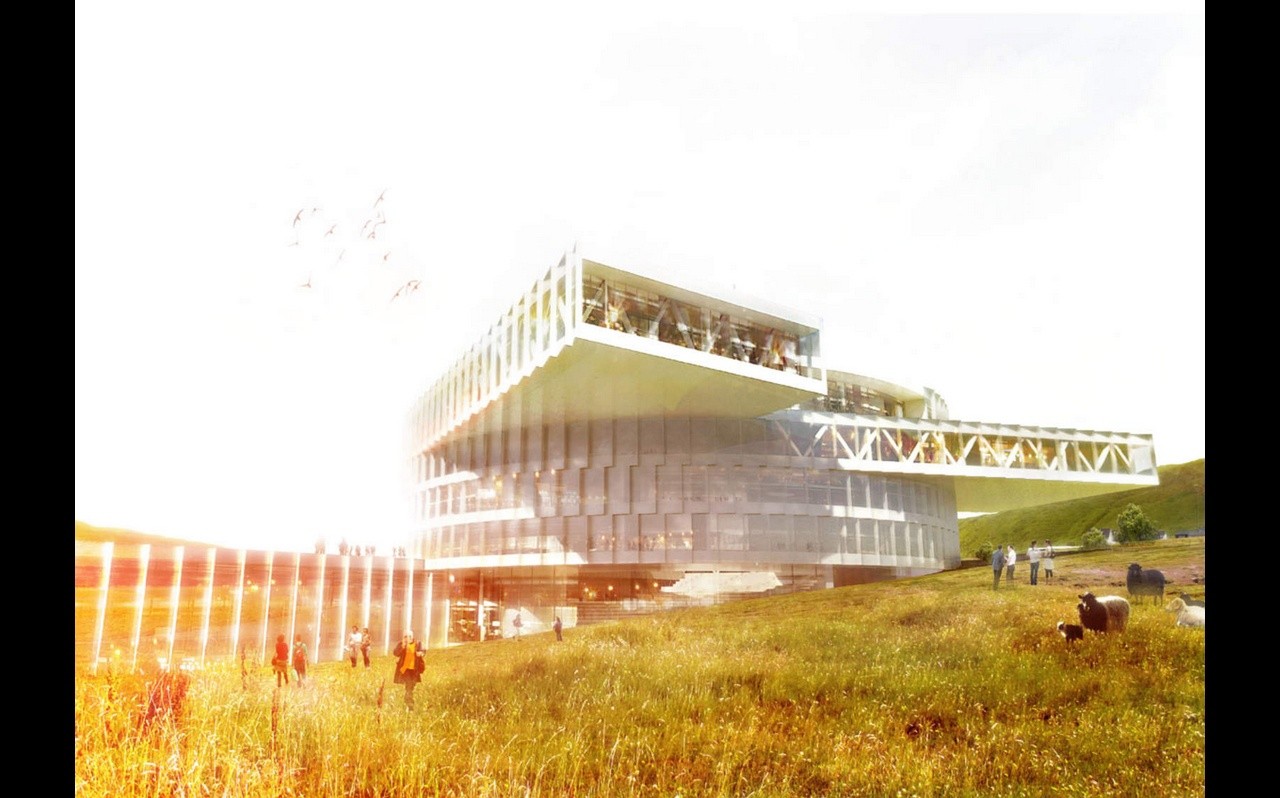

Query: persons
[1003,545,1017,581]
[1043,540,1053,584]
[293,635,310,681]
[348,626,370,667]
[991,545,1004,590]
[274,633,289,688]
[553,616,563,642]
[512,614,523,641]
[1027,541,1040,585]
[393,630,426,710]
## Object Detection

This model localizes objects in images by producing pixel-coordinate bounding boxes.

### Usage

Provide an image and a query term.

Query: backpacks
[294,642,304,661]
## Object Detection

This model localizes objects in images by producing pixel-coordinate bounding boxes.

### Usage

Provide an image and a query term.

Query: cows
[1056,563,1205,644]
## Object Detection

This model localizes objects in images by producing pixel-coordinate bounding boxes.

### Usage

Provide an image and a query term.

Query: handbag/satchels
[416,640,426,672]
